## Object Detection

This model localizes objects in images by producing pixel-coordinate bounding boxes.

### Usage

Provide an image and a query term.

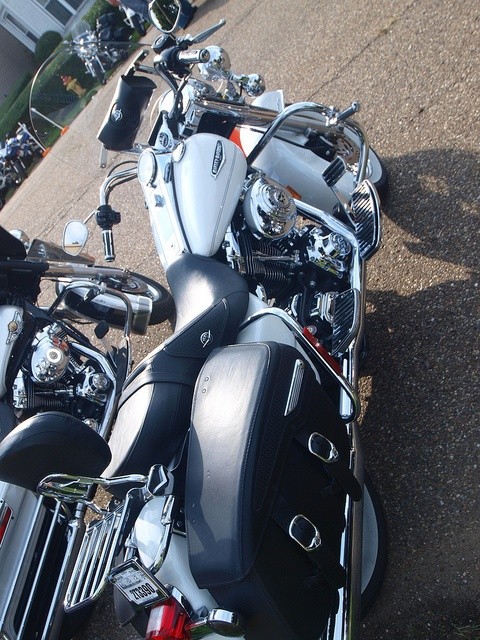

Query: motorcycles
[0,225,176,639]
[59,1,160,84]
[1,0,391,640]
[1,125,45,192]
